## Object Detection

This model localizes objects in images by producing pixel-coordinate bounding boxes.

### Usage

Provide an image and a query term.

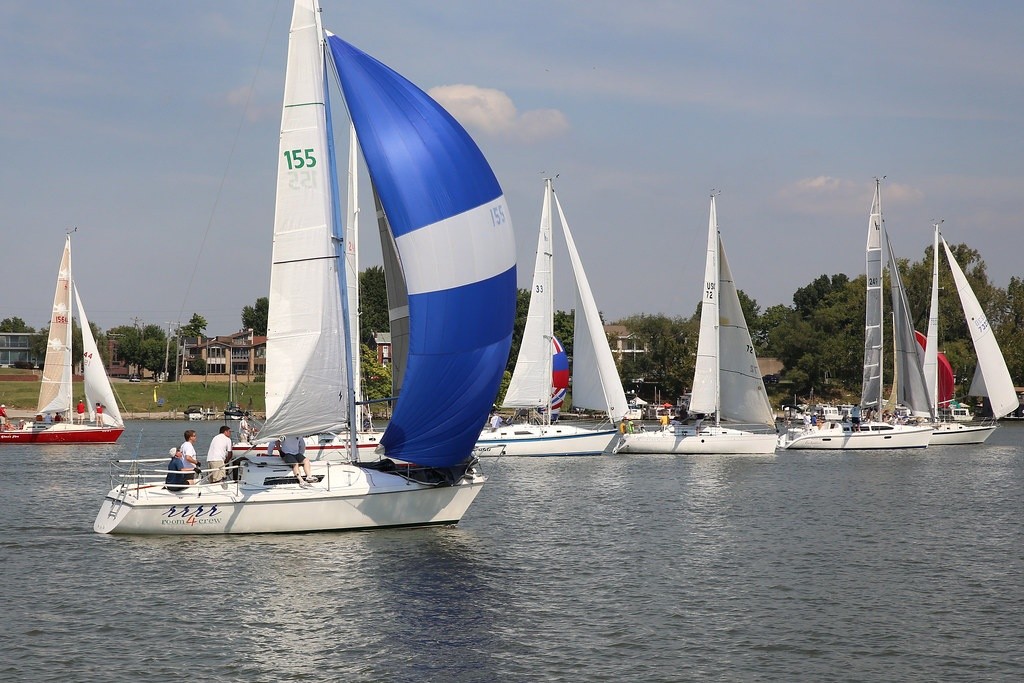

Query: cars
[129,374,140,382]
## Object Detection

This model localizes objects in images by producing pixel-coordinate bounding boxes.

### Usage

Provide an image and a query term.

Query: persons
[0,405,8,431]
[659,412,671,426]
[864,407,917,424]
[165,447,195,492]
[274,432,318,490]
[205,425,233,482]
[77,400,86,425]
[96,403,104,428]
[679,405,691,425]
[179,430,202,484]
[490,411,503,430]
[803,412,818,426]
[850,404,862,433]
[620,416,635,434]
[6,412,63,430]
[238,411,255,442]
[842,415,850,423]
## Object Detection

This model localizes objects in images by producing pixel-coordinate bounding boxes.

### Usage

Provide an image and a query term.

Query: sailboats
[472,171,631,456]
[922,218,1020,445]
[613,188,779,455]
[0,227,125,443]
[777,174,935,450]
[92,0,517,535]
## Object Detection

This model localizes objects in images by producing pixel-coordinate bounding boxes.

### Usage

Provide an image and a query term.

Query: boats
[783,402,975,424]
[224,411,243,420]
[183,409,201,419]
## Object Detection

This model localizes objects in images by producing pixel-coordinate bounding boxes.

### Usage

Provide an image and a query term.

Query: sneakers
[299,481,310,488]
[306,476,319,482]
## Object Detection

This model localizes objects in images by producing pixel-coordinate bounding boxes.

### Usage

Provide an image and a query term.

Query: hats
[1,405,5,407]
[170,448,177,456]
